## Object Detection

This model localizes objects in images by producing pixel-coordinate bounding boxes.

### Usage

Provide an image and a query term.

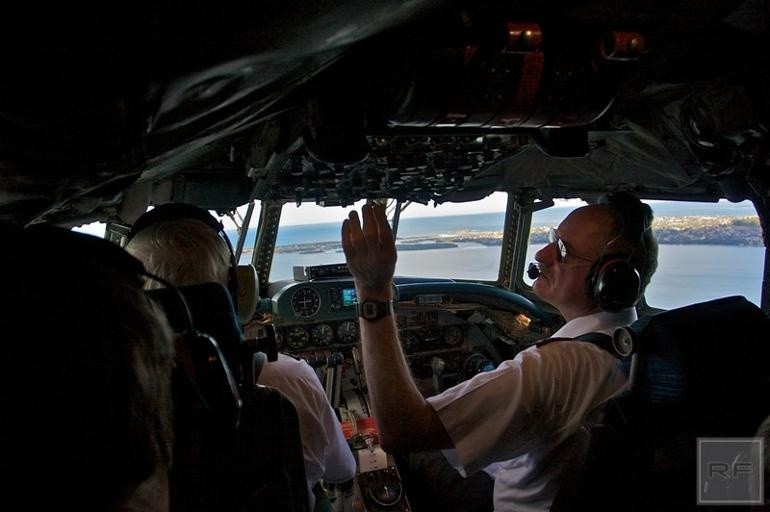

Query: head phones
[123,206,258,326]
[28,224,247,445]
[586,193,646,313]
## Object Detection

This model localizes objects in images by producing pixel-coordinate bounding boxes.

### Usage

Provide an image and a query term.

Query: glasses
[547,225,596,266]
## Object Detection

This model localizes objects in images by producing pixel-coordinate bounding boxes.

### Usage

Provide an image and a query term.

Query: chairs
[144,282,310,512]
[546,294,770,511]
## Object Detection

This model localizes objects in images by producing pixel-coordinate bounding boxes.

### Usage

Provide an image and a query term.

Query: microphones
[526,261,541,279]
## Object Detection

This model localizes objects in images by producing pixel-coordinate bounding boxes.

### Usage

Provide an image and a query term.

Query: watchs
[355,296,395,326]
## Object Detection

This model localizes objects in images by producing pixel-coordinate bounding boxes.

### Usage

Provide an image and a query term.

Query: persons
[0,260,178,511]
[338,195,662,512]
[123,215,361,512]
